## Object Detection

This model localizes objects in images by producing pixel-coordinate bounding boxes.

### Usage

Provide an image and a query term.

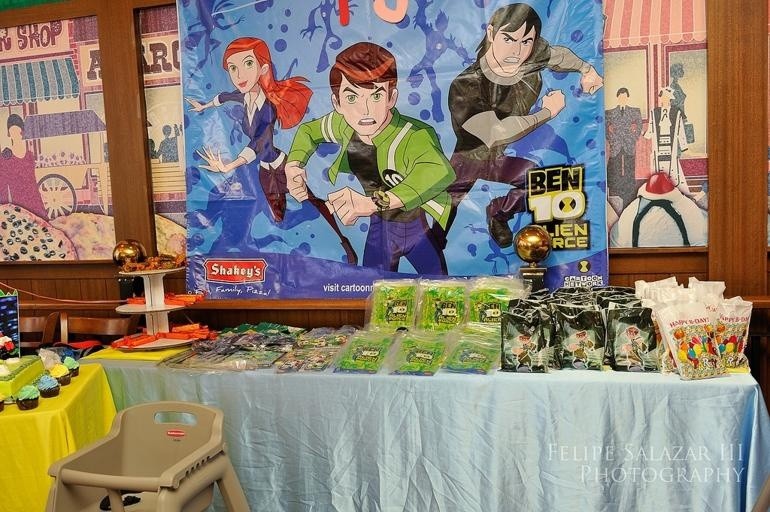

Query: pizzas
[49,213,117,262]
[0,203,79,262]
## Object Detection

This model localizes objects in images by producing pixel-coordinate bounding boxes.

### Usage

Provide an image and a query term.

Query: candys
[674,324,743,369]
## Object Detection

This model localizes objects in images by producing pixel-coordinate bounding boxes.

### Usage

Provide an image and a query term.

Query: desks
[0,363,117,512]
[77,330,770,512]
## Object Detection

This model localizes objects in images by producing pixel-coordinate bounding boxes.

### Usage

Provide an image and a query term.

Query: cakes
[612,170,709,248]
[39,376,59,397]
[64,357,79,377]
[51,364,70,387]
[15,385,39,410]
[1,354,45,403]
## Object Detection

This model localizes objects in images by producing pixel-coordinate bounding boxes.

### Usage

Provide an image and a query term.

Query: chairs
[46,400,250,512]
[60,312,131,348]
[19,312,59,347]
